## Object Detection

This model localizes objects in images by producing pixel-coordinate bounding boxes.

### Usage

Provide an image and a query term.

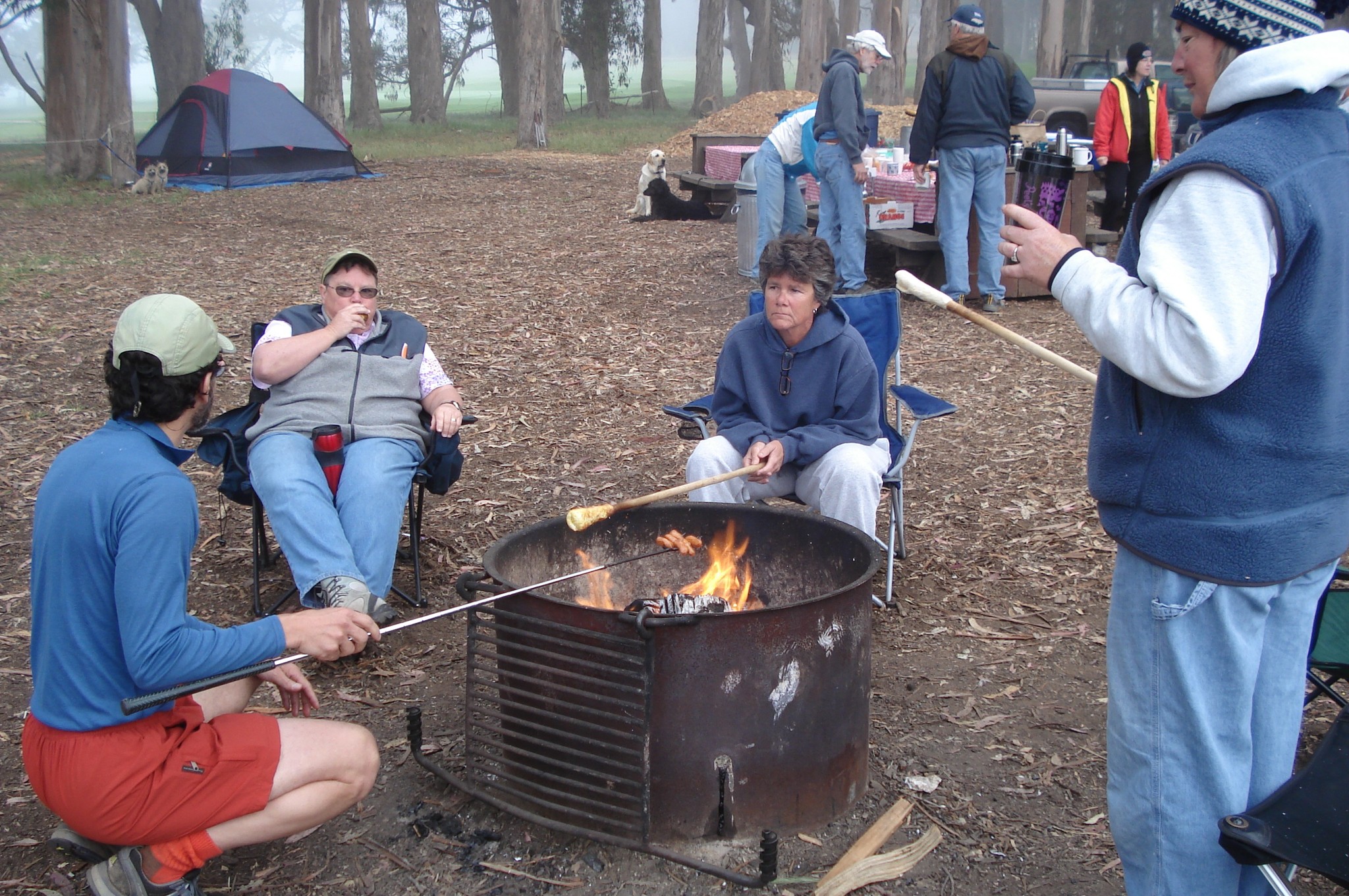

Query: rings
[451,418,457,422]
[1011,245,1020,263]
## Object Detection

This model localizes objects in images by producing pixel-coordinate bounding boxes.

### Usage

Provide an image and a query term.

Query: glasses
[947,24,953,31]
[324,283,379,299]
[779,350,794,396]
[197,360,230,379]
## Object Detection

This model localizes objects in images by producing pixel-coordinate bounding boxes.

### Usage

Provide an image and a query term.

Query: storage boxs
[863,197,914,230]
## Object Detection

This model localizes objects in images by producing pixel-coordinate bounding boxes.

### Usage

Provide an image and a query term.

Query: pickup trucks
[1031,49,1185,142]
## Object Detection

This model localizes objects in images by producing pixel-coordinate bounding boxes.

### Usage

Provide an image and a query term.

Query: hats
[942,5,987,27]
[113,293,237,377]
[320,249,379,290]
[1169,0,1349,52]
[847,30,892,60]
[1127,42,1152,65]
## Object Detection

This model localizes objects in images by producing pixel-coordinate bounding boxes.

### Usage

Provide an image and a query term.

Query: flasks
[310,424,345,496]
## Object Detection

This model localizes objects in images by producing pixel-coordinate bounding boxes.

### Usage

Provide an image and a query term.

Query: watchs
[439,400,463,412]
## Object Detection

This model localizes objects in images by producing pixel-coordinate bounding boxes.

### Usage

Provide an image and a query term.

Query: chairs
[1217,565,1349,896]
[662,288,961,609]
[184,322,478,618]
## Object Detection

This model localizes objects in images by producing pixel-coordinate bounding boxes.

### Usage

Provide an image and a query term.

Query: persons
[908,2,1036,314]
[1091,42,1172,257]
[1001,0,1349,895]
[752,100,819,284]
[685,233,893,541]
[246,249,467,669]
[22,292,382,895]
[813,28,893,295]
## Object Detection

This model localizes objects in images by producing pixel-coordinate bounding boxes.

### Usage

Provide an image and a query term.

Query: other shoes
[984,292,1000,312]
[955,294,967,306]
[749,278,761,285]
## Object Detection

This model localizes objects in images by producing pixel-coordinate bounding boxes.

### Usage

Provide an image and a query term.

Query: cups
[915,173,930,192]
[1069,144,1093,166]
[1036,142,1048,153]
[893,148,904,174]
[1006,147,1076,266]
[862,156,899,177]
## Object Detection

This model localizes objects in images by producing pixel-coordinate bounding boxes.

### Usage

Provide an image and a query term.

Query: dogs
[132,163,158,197]
[625,148,667,217]
[155,159,169,193]
[627,177,722,222]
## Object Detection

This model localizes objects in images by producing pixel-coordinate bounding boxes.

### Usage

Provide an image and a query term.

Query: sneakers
[49,822,123,864]
[313,576,399,628]
[834,283,877,294]
[86,844,208,896]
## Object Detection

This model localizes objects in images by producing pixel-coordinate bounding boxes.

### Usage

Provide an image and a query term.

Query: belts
[819,138,839,144]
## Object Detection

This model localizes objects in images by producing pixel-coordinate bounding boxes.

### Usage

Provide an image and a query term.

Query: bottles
[1056,127,1067,156]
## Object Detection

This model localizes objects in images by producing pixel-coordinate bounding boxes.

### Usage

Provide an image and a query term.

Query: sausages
[905,109,917,116]
[656,529,703,556]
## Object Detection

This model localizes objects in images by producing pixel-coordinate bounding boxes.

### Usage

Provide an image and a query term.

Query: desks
[802,168,937,223]
[704,146,761,181]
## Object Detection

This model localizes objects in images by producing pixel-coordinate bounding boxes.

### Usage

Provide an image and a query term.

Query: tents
[135,68,384,192]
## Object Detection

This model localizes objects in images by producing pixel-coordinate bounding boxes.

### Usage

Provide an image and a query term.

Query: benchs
[1086,226,1118,254]
[1086,190,1126,217]
[671,169,940,268]
